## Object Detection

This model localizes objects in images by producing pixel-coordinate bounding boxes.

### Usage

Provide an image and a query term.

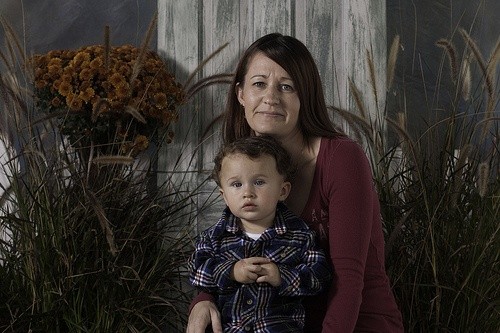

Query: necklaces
[284,146,305,205]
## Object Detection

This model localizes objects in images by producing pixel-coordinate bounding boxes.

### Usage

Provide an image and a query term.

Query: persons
[188,33,404,333]
[188,136,332,333]
